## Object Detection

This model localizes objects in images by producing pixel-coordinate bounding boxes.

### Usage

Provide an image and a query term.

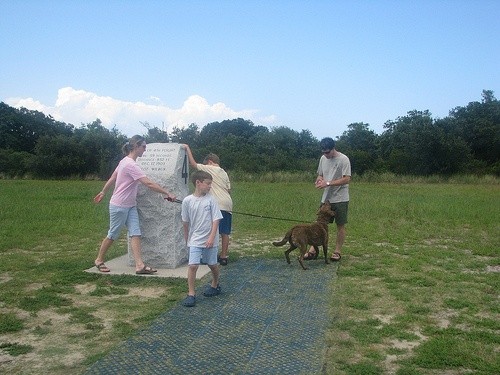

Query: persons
[181,170,223,307]
[182,143,233,264]
[298,138,351,260]
[93,135,176,274]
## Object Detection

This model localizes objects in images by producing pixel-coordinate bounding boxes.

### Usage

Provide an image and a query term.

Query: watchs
[326,181,330,187]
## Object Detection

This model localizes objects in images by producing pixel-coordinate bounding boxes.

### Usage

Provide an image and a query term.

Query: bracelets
[100,192,105,196]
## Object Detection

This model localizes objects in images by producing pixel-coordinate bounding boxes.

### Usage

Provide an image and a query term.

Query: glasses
[321,149,331,154]
[141,144,146,149]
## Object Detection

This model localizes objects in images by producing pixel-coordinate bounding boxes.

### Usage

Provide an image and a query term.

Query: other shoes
[184,295,196,306]
[218,255,227,264]
[205,285,220,296]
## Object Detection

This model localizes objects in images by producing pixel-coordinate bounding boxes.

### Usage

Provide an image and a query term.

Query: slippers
[96,263,110,272]
[136,266,157,273]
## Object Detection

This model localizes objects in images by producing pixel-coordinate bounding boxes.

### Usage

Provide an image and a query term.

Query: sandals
[297,252,316,259]
[330,253,341,260]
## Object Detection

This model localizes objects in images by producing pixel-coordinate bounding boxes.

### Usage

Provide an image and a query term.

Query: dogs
[272,203,336,269]
